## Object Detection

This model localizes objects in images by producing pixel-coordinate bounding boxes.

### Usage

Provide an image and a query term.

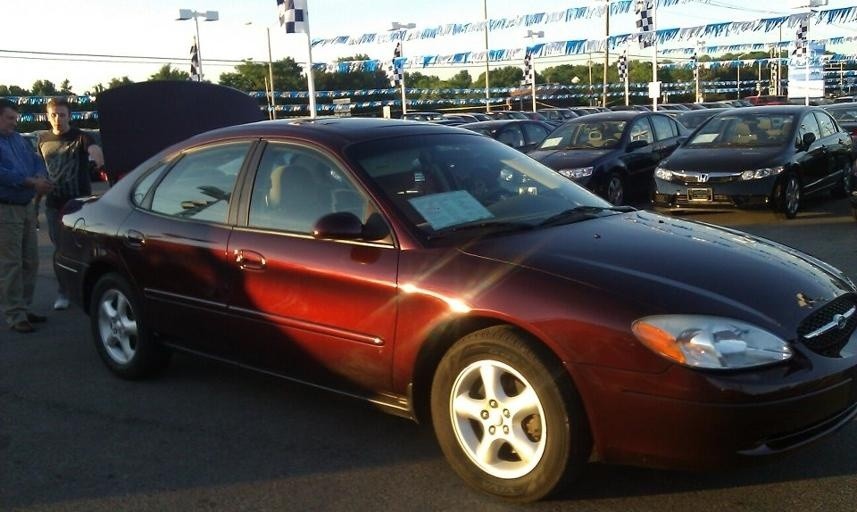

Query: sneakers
[28,312,46,323]
[54,294,69,309]
[13,320,33,332]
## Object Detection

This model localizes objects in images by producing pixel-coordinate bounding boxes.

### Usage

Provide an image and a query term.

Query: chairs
[266,165,324,227]
[728,122,760,145]
[754,119,792,139]
[584,130,604,146]
[380,159,422,203]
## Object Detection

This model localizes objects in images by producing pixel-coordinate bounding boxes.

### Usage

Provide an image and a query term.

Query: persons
[28,95,104,310]
[0,97,55,333]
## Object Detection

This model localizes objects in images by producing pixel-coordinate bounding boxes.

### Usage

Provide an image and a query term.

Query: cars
[400,94,857,155]
[649,103,855,219]
[13,125,107,185]
[54,77,857,508]
[494,110,697,205]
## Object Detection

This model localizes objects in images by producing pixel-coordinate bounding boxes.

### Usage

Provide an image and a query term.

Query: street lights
[582,1,847,109]
[247,18,279,122]
[522,28,547,113]
[387,19,418,121]
[176,5,222,83]
